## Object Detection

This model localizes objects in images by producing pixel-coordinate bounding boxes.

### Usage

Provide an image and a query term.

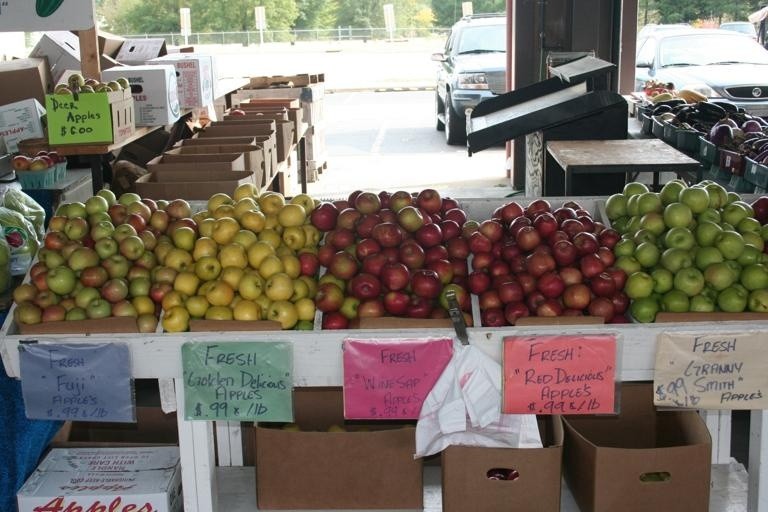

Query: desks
[543,139,701,196]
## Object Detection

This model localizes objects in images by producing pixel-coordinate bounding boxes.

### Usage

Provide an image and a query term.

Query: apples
[231,108,246,116]
[10,150,61,172]
[0,178,768,333]
[55,72,129,93]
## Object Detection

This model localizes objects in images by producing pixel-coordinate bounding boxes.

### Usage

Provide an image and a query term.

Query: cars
[635,22,768,122]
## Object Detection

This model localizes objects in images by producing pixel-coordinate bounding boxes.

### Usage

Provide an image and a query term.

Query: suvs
[430,15,510,146]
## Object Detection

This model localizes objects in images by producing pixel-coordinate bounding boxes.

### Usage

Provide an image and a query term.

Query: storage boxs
[1,30,215,190]
[637,106,768,189]
[135,97,303,200]
[562,383,712,512]
[440,414,565,512]
[51,407,179,447]
[16,446,184,512]
[255,389,424,510]
